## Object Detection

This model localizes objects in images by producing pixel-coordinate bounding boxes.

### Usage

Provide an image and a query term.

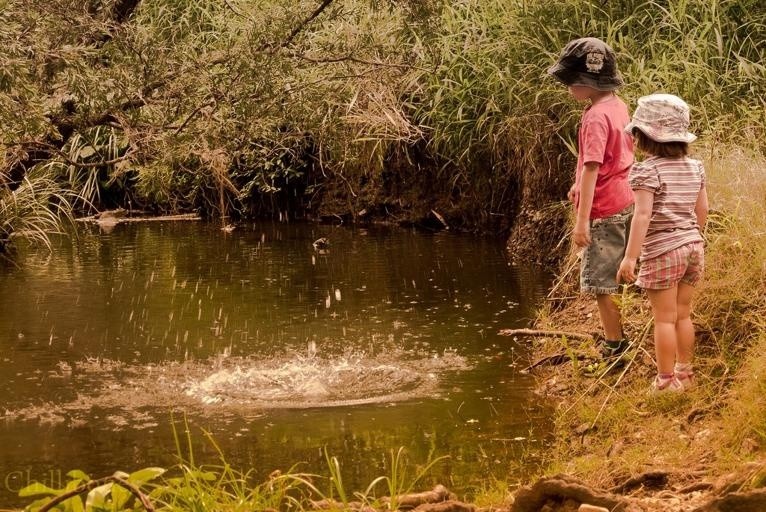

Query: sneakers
[601,338,625,367]
[647,367,695,392]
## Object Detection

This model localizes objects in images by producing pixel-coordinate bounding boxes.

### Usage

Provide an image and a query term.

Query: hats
[624,94,698,143]
[547,37,624,91]
